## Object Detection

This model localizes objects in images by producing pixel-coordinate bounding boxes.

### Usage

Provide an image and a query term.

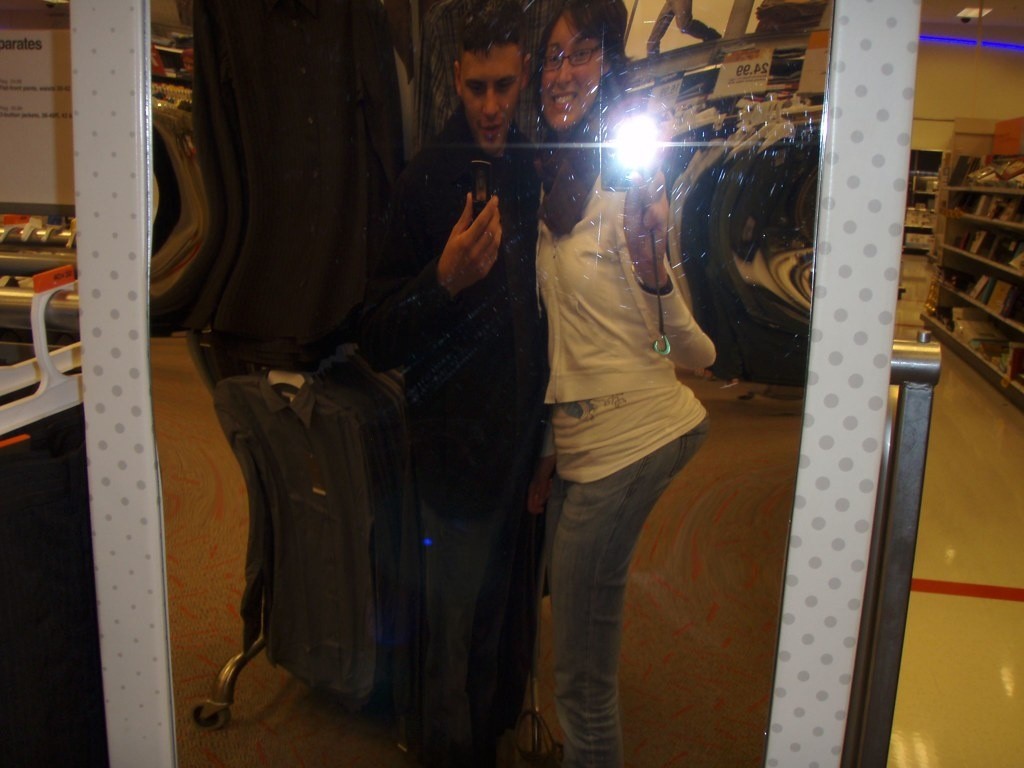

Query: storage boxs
[907,207,934,249]
[946,115,1024,186]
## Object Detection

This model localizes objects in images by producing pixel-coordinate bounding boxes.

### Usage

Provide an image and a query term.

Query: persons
[646,0,723,58]
[536,0,717,763]
[350,0,551,768]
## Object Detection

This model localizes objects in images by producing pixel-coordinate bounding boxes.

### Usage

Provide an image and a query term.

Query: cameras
[598,148,641,192]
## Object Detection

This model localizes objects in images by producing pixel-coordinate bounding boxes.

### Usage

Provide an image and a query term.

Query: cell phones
[471,160,491,222]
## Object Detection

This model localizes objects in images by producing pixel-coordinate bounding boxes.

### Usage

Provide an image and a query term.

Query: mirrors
[69,0,922,767]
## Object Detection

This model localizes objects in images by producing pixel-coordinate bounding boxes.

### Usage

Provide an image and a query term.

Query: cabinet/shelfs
[903,191,937,252]
[920,186,1024,410]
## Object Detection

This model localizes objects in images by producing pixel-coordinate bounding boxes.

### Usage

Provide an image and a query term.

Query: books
[936,150,1024,386]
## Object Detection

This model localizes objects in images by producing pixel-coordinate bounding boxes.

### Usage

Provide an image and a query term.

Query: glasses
[538,42,608,71]
